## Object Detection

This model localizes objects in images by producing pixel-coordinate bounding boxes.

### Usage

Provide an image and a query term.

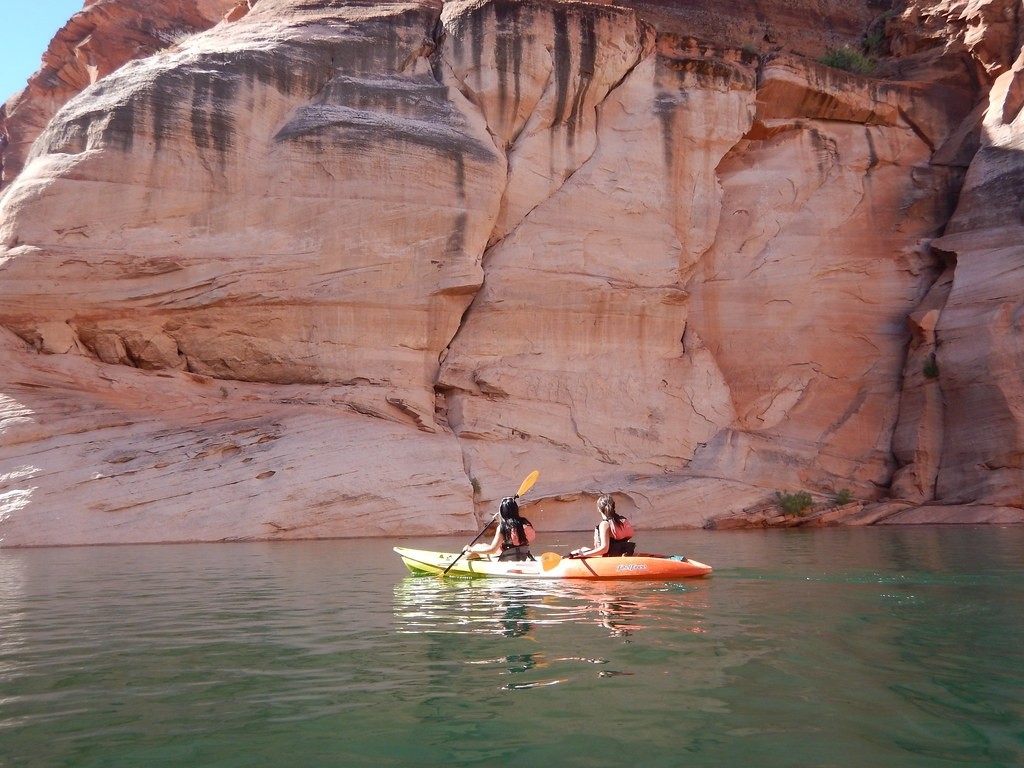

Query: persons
[573,494,633,558]
[463,494,537,561]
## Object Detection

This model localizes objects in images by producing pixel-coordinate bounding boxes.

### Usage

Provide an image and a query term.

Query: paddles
[440,470,540,576]
[542,549,594,571]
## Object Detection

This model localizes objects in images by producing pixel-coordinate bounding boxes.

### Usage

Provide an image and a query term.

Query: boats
[393,547,713,582]
